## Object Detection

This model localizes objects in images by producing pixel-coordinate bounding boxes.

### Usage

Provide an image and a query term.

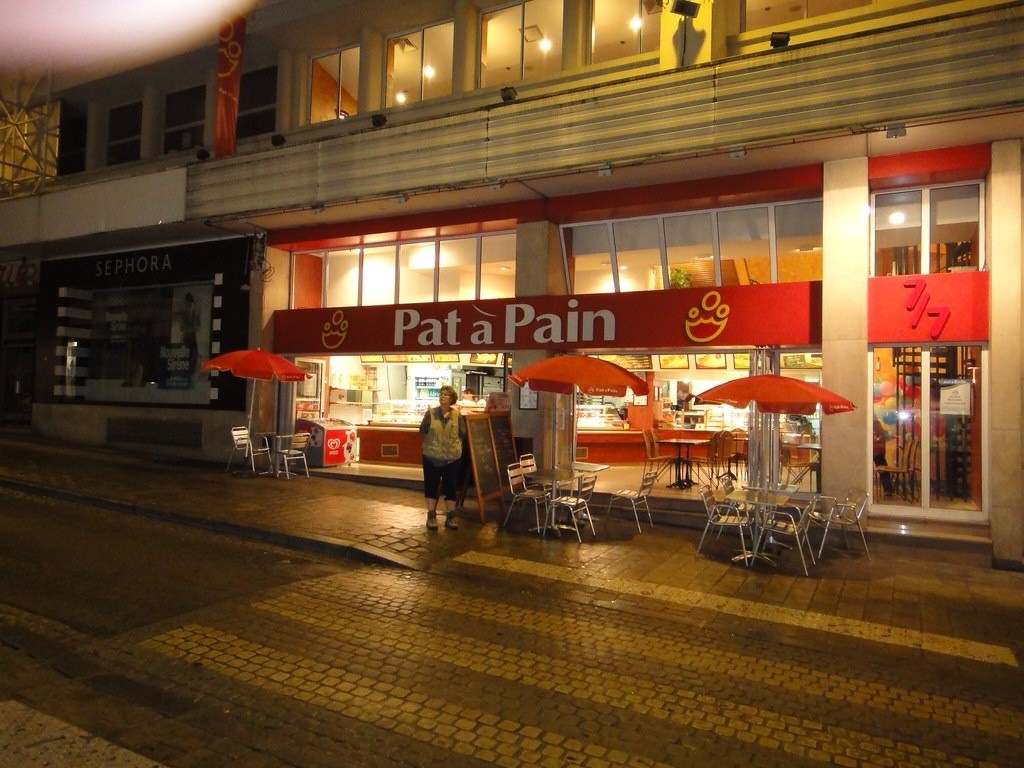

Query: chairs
[501,427,918,578]
[226,426,311,480]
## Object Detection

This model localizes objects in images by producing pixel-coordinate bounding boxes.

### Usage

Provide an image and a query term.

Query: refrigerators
[295,418,358,468]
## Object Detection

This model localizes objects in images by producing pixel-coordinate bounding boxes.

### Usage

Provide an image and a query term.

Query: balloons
[873,365,948,454]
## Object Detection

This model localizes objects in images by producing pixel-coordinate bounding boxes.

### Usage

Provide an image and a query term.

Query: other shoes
[426,518,438,529]
[445,518,459,528]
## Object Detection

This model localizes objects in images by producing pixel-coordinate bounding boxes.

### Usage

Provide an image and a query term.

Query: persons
[872,412,895,499]
[419,386,466,530]
[461,390,479,404]
[179,292,200,376]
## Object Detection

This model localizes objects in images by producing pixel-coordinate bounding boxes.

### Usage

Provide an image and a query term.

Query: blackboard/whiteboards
[465,413,504,502]
[485,410,520,493]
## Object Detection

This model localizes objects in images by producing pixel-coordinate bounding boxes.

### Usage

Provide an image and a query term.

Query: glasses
[439,393,450,396]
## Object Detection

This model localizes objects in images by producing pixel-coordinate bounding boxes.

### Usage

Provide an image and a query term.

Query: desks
[725,491,790,566]
[555,462,609,521]
[797,444,822,493]
[252,432,295,476]
[656,438,710,490]
[525,468,584,532]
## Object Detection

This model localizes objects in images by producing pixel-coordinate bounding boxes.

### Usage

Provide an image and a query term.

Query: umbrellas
[694,374,857,553]
[507,355,650,525]
[200,348,311,471]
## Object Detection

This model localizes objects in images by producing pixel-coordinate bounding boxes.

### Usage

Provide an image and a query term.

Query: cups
[690,422,696,428]
